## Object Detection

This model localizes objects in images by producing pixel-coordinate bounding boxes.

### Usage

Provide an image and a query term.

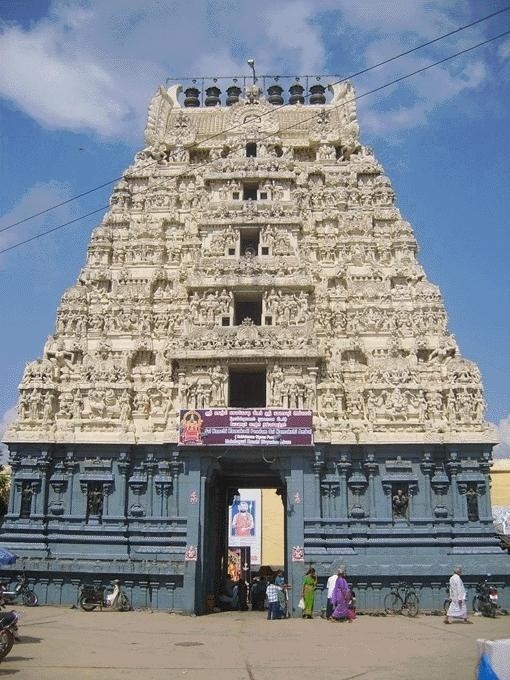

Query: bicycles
[384,584,419,616]
[444,586,452,611]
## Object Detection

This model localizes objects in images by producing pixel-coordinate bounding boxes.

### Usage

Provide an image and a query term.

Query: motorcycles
[0,566,38,606]
[78,579,130,612]
[472,573,498,617]
[0,610,21,660]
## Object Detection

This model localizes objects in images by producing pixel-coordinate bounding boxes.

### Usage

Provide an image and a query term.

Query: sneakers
[303,614,312,619]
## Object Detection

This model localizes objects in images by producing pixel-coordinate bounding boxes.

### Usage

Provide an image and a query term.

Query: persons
[392,489,407,514]
[89,487,103,515]
[330,568,357,622]
[301,568,318,619]
[348,585,355,597]
[233,569,288,620]
[445,566,474,624]
[15,107,490,434]
[233,502,255,538]
[324,574,339,620]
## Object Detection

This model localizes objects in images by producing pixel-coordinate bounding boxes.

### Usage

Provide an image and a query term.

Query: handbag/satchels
[298,597,305,610]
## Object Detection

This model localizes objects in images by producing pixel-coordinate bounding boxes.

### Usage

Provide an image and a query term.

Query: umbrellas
[0,545,19,569]
